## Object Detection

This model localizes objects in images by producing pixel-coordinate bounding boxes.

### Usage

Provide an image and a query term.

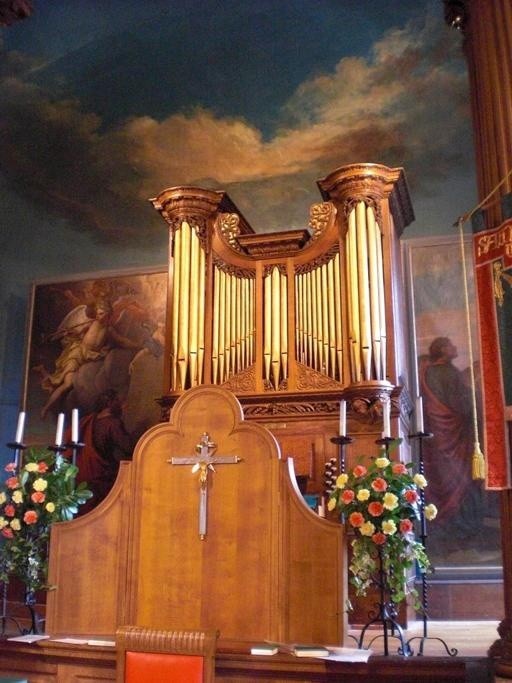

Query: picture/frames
[400,233,507,582]
[17,264,170,516]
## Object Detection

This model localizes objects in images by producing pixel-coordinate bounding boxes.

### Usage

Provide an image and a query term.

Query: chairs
[114,623,220,682]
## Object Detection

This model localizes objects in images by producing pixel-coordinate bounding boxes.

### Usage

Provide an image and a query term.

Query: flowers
[327,458,438,615]
[0,450,95,594]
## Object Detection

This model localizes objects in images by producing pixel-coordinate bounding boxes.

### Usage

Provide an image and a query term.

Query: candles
[415,396,425,433]
[16,411,27,443]
[56,413,65,445]
[383,403,392,438]
[72,408,82,442]
[339,399,346,435]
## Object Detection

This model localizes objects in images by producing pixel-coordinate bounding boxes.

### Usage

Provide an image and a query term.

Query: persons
[31,294,145,420]
[126,315,165,371]
[68,388,134,520]
[413,331,480,537]
